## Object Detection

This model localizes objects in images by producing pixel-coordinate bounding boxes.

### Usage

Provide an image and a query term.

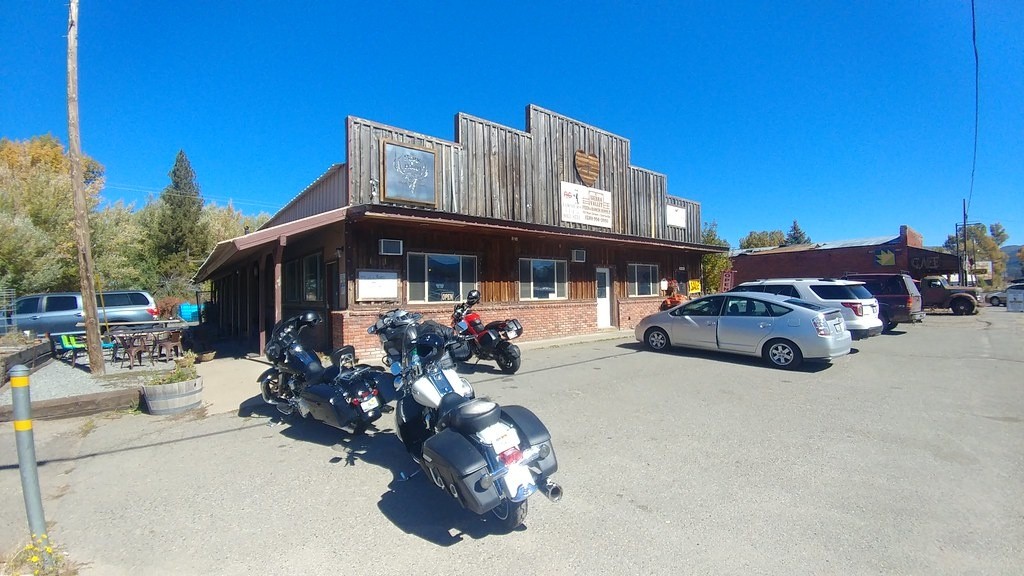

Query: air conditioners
[378,239,403,256]
[571,250,586,262]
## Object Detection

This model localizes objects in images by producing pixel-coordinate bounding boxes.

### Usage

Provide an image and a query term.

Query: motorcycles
[257,312,394,432]
[388,326,562,531]
[366,297,431,367]
[449,302,523,375]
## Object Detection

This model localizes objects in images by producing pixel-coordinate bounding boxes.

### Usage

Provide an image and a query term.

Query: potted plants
[141,349,203,416]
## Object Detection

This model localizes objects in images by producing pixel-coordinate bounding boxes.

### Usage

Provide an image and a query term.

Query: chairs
[57,334,87,368]
[109,329,184,370]
[730,304,740,314]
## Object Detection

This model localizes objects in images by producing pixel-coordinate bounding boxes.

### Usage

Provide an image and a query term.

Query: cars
[0,290,160,348]
[635,291,852,370]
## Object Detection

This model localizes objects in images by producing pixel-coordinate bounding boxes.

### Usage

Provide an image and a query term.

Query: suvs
[984,283,1024,307]
[838,272,927,334]
[717,277,883,339]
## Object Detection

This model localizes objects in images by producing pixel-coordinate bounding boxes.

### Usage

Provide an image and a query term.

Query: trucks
[914,275,986,318]
